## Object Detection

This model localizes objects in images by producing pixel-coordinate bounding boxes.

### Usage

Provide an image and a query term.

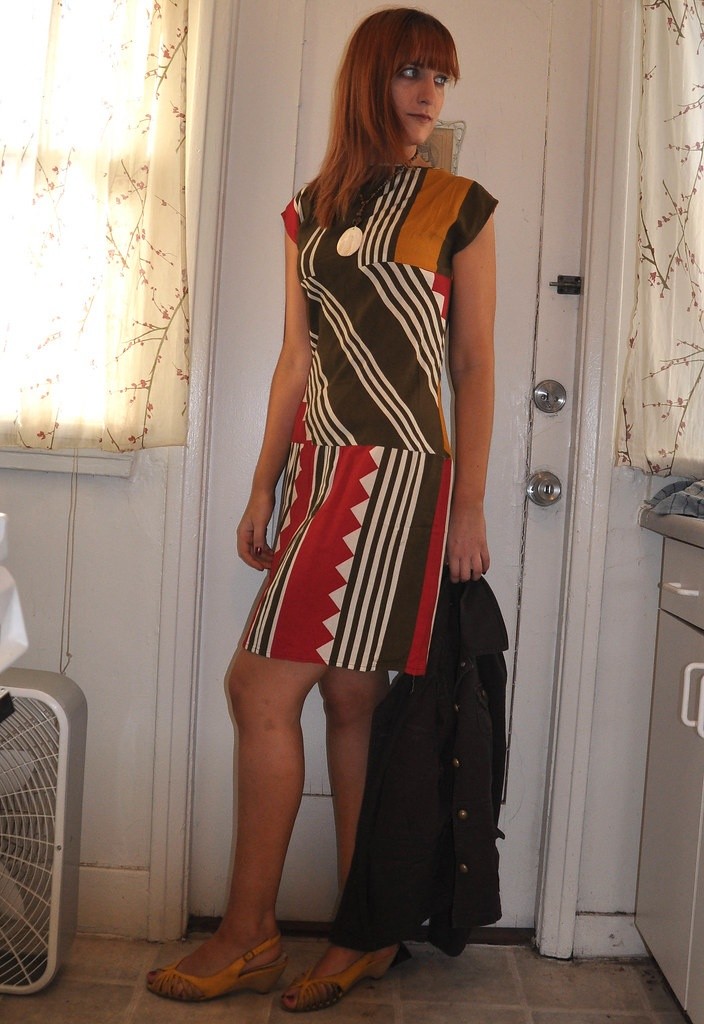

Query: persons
[143,8,499,1010]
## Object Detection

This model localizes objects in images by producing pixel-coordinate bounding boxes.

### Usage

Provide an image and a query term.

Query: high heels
[146,931,287,1001]
[280,941,400,1013]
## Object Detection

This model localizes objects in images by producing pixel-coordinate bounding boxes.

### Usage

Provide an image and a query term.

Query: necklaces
[337,149,420,256]
